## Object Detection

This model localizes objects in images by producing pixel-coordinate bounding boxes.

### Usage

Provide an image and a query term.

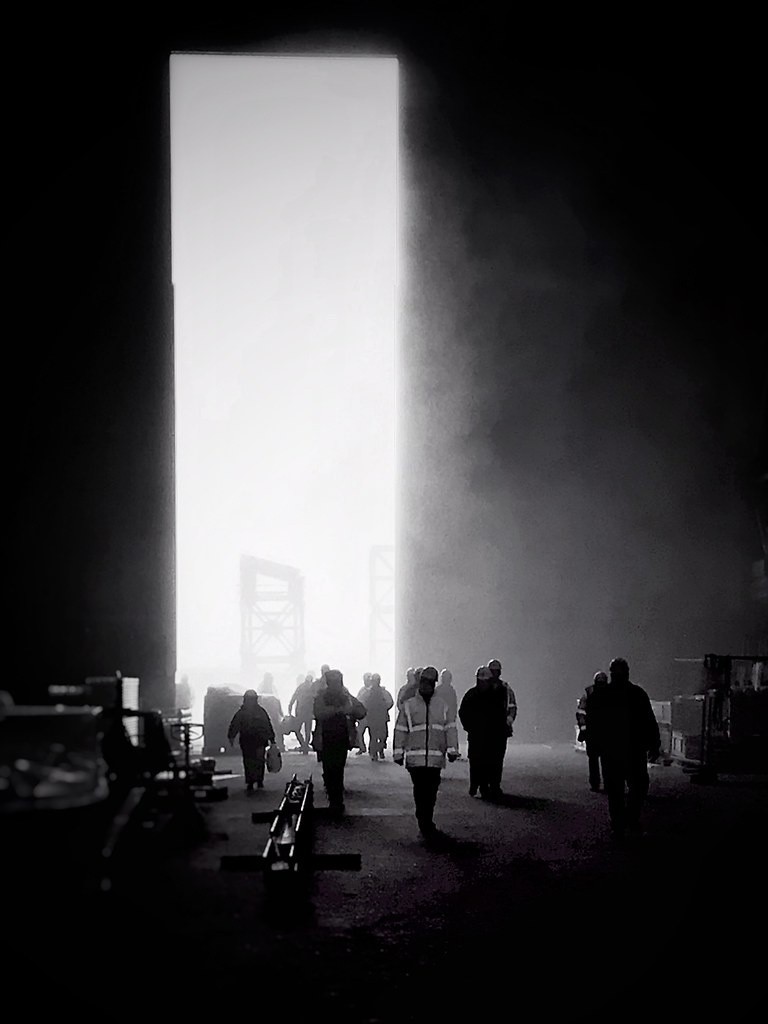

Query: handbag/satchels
[267,743,282,773]
[279,715,297,735]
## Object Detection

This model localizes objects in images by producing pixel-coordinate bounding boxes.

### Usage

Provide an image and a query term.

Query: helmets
[441,670,452,681]
[306,675,313,682]
[488,660,503,669]
[477,667,493,680]
[608,657,629,673]
[407,668,423,682]
[370,673,381,682]
[593,672,608,682]
[321,665,330,672]
[421,667,438,681]
[364,673,373,683]
[244,690,258,698]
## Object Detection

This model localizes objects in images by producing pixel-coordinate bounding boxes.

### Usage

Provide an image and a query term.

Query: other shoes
[417,831,430,845]
[610,829,625,842]
[247,786,253,795]
[630,823,642,839]
[257,784,264,788]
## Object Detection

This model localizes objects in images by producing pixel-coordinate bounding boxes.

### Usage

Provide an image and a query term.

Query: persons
[171,659,518,799]
[574,670,618,793]
[311,670,368,813]
[594,656,662,833]
[393,666,459,841]
